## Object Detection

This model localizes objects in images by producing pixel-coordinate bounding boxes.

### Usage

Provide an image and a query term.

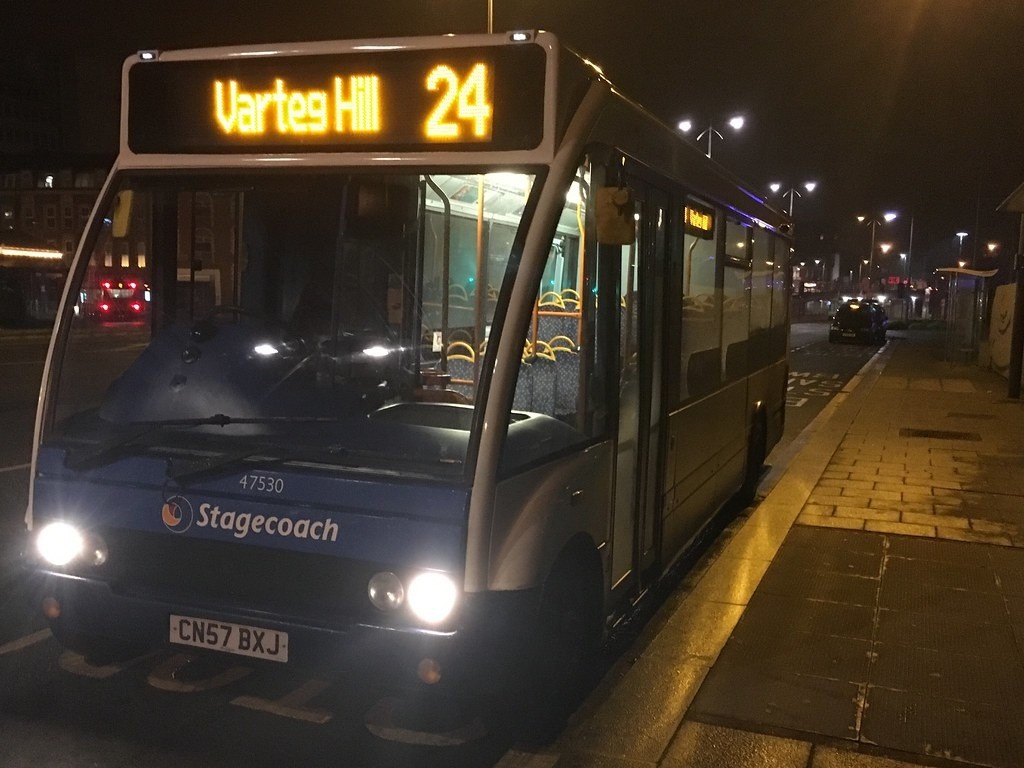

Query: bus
[97,276,149,321]
[22,30,797,753]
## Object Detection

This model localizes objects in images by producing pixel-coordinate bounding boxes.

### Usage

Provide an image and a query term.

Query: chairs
[409,277,533,327]
[425,289,753,428]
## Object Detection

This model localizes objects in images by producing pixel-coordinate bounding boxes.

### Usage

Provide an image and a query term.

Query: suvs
[828,298,887,345]
